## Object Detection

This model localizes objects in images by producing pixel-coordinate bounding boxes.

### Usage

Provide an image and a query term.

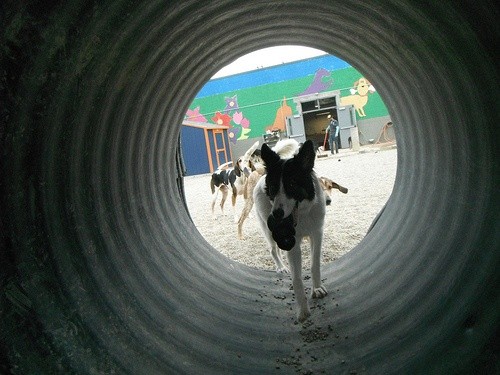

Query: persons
[324,114,341,155]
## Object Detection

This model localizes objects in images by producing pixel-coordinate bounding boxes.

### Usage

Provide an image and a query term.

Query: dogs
[251,140,329,323]
[235,136,349,242]
[210,155,256,217]
